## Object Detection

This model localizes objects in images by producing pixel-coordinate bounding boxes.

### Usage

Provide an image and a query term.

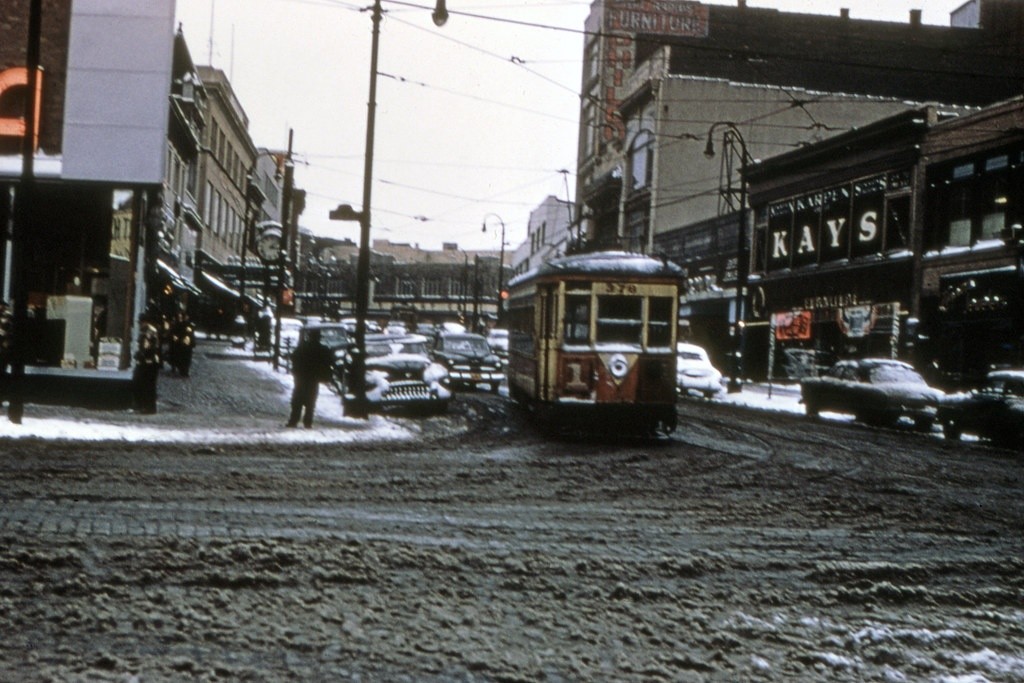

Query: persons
[135,313,160,413]
[285,328,334,428]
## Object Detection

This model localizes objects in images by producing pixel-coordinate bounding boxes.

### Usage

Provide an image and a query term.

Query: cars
[798,356,946,430]
[340,337,452,415]
[676,343,725,399]
[268,311,511,376]
[936,371,1024,447]
[428,332,504,392]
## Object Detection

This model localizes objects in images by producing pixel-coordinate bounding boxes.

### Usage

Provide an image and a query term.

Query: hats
[307,328,323,337]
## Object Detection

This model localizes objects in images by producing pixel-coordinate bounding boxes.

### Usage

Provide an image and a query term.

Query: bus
[509,245,690,435]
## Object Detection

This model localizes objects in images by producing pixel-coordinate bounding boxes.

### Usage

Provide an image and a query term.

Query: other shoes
[286,421,297,428]
[304,422,312,429]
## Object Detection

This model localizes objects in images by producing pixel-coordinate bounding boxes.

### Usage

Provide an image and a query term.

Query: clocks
[257,235,281,261]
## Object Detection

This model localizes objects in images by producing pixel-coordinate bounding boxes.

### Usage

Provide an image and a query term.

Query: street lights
[700,120,748,393]
[328,0,450,417]
[239,152,294,291]
[454,213,504,334]
